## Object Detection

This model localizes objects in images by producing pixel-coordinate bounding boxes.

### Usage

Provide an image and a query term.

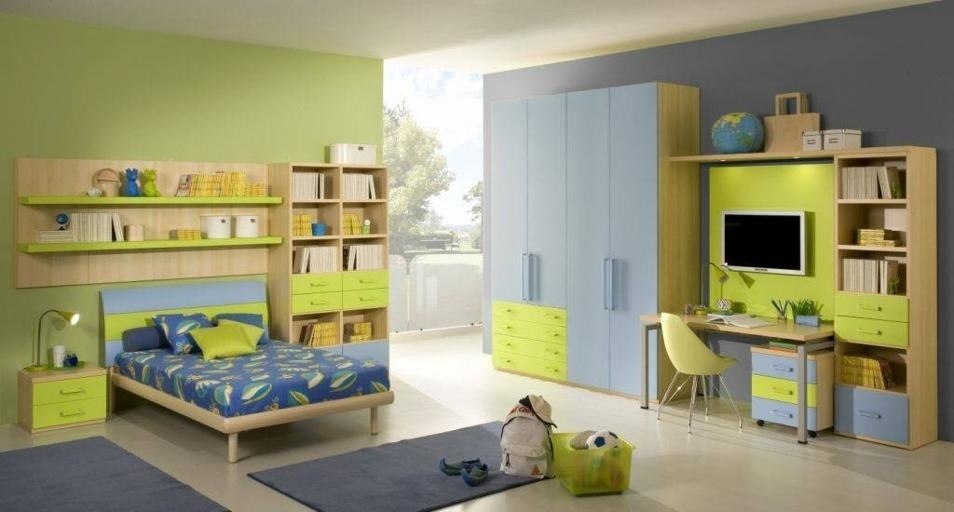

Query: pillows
[165,313,213,355]
[215,318,264,349]
[216,312,268,345]
[151,314,184,348]
[247,420,548,512]
[121,326,171,352]
[188,324,256,362]
[100,279,394,463]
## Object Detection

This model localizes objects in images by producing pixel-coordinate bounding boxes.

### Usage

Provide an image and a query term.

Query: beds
[100,279,394,463]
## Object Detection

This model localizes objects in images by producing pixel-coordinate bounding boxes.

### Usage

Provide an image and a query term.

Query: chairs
[656,312,743,434]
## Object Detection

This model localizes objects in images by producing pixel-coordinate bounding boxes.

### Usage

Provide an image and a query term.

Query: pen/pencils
[772,298,788,313]
[789,297,823,313]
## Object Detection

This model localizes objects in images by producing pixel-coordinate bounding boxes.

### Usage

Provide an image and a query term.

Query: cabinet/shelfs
[750,341,853,439]
[568,81,701,404]
[16,362,108,435]
[834,145,938,451]
[267,161,391,374]
[15,158,269,288]
[491,90,568,386]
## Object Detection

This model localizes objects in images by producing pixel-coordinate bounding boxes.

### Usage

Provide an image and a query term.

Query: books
[289,168,384,346]
[31,210,202,244]
[836,164,905,392]
[704,313,768,329]
[169,168,267,198]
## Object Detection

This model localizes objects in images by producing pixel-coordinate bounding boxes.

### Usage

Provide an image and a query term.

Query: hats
[519,392,560,429]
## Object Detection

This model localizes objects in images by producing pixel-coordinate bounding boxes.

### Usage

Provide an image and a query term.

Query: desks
[640,313,835,444]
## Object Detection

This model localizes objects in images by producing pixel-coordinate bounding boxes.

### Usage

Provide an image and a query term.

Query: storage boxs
[547,430,635,498]
[330,143,377,166]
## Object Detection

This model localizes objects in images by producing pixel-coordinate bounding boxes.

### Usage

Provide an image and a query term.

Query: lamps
[696,262,729,320]
[25,310,79,371]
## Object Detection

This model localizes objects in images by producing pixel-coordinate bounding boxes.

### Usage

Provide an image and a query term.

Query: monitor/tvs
[721,211,806,276]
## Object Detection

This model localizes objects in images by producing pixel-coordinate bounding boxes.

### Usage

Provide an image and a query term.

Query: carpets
[0,436,232,512]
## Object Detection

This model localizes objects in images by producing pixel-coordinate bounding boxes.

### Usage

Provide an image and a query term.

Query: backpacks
[498,401,557,479]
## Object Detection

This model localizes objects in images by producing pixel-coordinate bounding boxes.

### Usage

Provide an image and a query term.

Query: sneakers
[438,455,483,476]
[460,462,490,488]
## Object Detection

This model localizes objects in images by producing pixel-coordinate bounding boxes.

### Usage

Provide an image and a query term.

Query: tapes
[696,310,708,315]
